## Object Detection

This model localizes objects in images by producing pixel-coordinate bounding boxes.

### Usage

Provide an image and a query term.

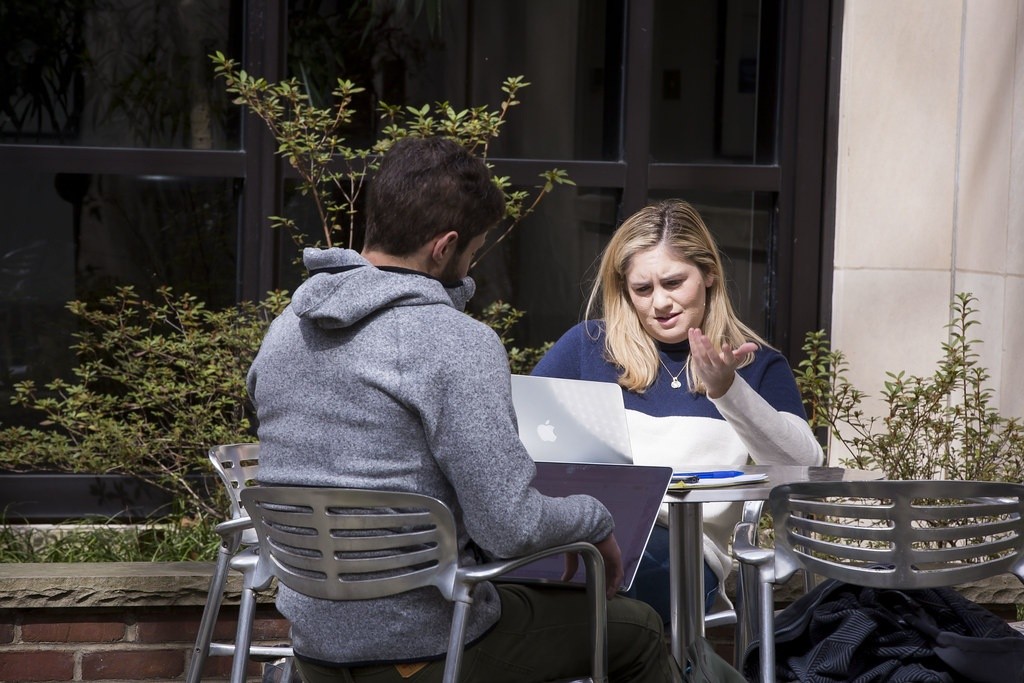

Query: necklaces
[657,349,692,389]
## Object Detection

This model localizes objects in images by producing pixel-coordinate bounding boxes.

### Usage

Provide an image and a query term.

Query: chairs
[184,443,259,683]
[229,486,610,683]
[732,481,1024,683]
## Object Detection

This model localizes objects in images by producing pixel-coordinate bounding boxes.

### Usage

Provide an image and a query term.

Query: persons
[527,199,826,619]
[246,140,666,683]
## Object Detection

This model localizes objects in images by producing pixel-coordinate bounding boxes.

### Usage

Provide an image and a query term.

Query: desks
[661,466,887,683]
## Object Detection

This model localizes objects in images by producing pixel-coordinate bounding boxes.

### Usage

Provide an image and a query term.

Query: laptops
[479,463,673,592]
[510,373,634,467]
